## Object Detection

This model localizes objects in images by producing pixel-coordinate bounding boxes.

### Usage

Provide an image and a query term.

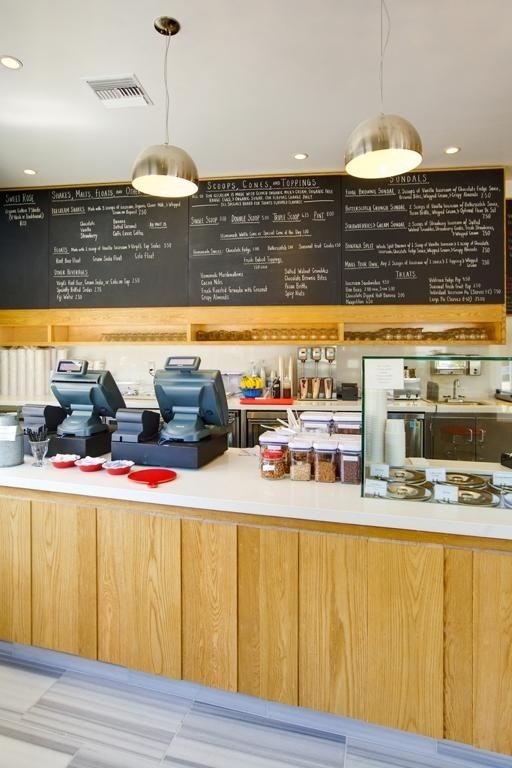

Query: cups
[29,439,50,466]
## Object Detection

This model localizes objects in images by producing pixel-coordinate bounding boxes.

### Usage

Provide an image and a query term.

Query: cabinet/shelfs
[0,306,506,345]
[427,413,512,462]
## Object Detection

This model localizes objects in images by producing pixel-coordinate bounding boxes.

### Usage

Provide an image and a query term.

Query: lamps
[343,0,423,179]
[131,18,199,199]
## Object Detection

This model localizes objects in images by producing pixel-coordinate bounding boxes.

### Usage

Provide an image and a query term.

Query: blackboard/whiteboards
[0,165,506,319]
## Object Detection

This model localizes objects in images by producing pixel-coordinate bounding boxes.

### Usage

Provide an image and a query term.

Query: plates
[239,388,262,397]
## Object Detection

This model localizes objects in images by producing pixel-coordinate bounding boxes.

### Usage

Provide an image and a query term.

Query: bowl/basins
[75,459,106,472]
[49,454,80,468]
[102,460,135,474]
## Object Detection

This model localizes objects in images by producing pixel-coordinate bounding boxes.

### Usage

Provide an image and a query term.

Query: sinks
[438,400,488,405]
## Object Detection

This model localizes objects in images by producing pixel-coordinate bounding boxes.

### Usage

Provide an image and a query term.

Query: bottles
[259,434,289,480]
[338,442,362,484]
[288,440,313,480]
[313,443,338,483]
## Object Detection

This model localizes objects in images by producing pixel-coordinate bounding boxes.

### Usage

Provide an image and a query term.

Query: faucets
[453,379,461,398]
